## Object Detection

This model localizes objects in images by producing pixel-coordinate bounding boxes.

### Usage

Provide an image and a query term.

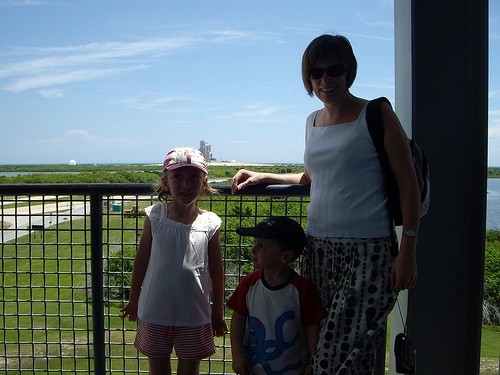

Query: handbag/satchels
[366,97,430,226]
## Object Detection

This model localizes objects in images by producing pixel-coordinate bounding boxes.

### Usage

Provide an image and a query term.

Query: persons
[120,146,229,375]
[226,214,329,375]
[232,36,420,375]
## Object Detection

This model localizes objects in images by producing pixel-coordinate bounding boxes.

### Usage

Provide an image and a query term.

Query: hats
[162,147,209,175]
[235,216,306,250]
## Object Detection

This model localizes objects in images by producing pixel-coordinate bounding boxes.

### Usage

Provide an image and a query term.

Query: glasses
[307,63,346,79]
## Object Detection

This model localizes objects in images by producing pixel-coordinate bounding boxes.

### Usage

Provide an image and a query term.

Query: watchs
[402,228,418,238]
